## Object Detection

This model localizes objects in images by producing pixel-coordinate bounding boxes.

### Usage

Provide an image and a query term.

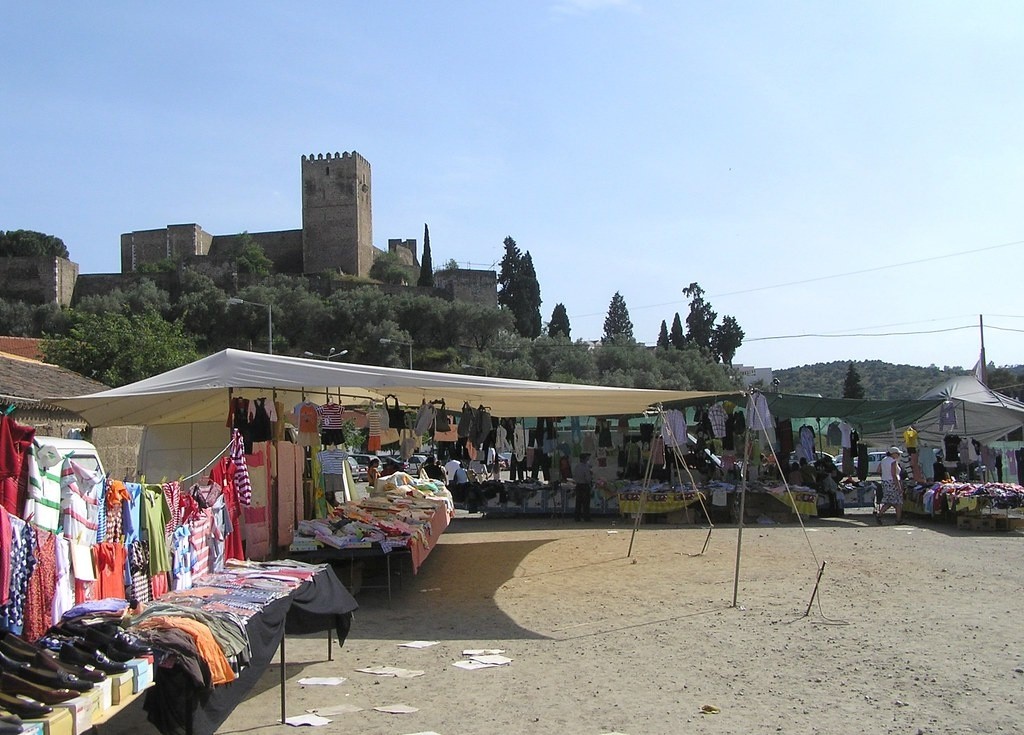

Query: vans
[30,436,106,478]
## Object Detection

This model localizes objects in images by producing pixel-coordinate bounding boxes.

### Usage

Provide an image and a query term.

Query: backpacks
[431,400,450,432]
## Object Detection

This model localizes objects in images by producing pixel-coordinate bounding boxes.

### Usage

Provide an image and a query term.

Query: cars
[849,451,888,478]
[347,457,360,483]
[787,451,835,471]
[349,454,383,481]
[376,456,411,476]
[832,455,843,473]
[499,453,513,468]
[498,454,509,471]
[395,455,428,474]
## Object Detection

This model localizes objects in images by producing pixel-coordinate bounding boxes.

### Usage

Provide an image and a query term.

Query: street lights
[459,363,487,378]
[304,348,348,363]
[380,337,413,370]
[229,297,274,355]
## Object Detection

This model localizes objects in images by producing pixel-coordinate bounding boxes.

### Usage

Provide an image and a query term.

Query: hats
[890,447,904,454]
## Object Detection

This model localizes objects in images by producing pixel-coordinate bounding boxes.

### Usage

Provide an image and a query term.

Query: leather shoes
[0,619,152,735]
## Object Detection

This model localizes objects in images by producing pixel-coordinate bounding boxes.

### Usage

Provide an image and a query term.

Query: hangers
[304,397,311,404]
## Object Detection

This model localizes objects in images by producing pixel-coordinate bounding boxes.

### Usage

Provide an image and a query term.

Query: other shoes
[575,516,581,521]
[584,516,590,521]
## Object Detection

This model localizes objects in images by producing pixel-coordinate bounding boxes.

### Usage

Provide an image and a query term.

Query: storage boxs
[81,687,103,721]
[18,722,45,735]
[105,669,134,706]
[666,509,695,526]
[333,562,363,592]
[731,494,791,524]
[124,658,147,693]
[55,697,92,735]
[956,514,1024,531]
[92,678,113,711]
[140,654,154,684]
[22,707,73,735]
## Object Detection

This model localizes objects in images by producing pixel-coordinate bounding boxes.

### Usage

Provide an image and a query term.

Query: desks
[447,484,1024,531]
[91,561,359,735]
[279,496,451,609]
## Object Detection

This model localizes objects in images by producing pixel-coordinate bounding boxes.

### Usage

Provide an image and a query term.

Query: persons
[419,456,446,482]
[469,459,506,482]
[875,447,907,525]
[574,453,594,522]
[445,459,477,484]
[789,457,815,489]
[367,459,381,486]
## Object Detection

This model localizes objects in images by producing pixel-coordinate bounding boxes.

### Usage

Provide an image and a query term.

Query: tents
[41,348,752,607]
[752,376,1024,459]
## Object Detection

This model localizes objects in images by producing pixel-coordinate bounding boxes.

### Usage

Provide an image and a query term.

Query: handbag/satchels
[399,428,415,458]
[414,398,436,436]
[366,398,390,430]
[385,394,406,429]
[458,402,491,442]
[378,426,399,445]
[435,415,458,441]
[398,429,422,448]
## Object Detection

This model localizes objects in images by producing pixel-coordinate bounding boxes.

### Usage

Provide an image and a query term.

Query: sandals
[875,514,882,525]
[895,519,906,525]
